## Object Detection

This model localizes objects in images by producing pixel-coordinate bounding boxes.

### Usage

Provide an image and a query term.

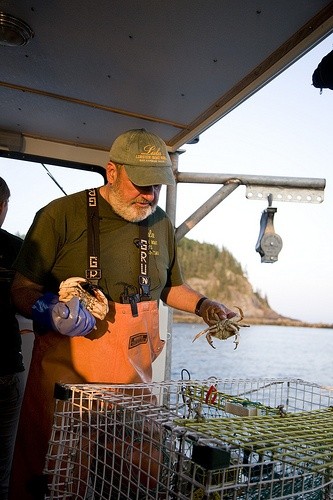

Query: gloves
[31,294,95,336]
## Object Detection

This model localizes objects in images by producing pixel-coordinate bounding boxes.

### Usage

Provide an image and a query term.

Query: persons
[0,177,24,500]
[7,128,240,500]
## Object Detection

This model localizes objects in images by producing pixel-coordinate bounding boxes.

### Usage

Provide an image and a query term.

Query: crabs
[191,305,251,350]
[57,275,111,321]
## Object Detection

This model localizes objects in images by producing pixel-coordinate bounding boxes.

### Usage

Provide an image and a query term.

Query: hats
[109,128,175,186]
